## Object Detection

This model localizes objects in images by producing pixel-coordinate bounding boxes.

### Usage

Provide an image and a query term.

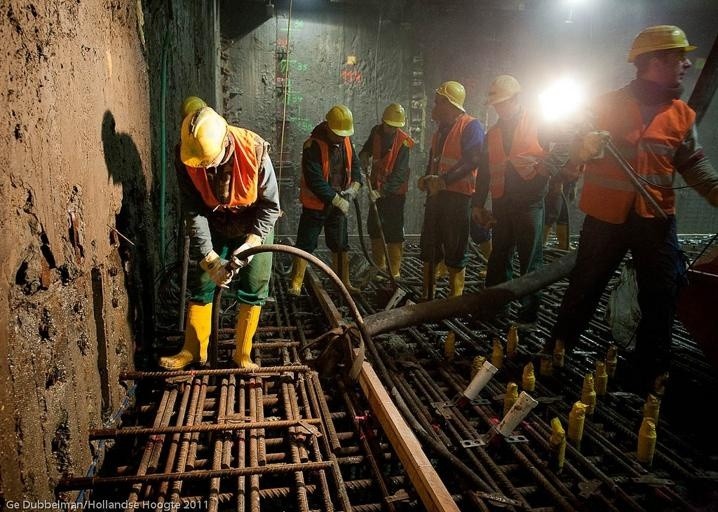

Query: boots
[555,221,569,251]
[287,254,309,297]
[234,303,262,368]
[367,228,386,275]
[417,261,440,303]
[446,266,466,299]
[479,239,493,278]
[373,242,402,283]
[331,250,362,295]
[158,301,213,370]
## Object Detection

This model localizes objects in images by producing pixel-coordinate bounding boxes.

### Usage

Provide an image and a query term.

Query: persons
[157,108,280,370]
[472,79,552,329]
[359,104,414,283]
[173,96,207,161]
[539,115,581,252]
[416,81,480,306]
[287,104,361,297]
[543,26,717,395]
[429,107,492,280]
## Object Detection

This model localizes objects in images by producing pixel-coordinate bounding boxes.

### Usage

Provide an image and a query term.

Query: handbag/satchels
[418,176,427,190]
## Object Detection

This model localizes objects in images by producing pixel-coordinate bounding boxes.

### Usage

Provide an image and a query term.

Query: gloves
[331,192,350,218]
[427,174,447,193]
[341,181,362,201]
[229,233,263,275]
[199,250,234,289]
[581,130,610,158]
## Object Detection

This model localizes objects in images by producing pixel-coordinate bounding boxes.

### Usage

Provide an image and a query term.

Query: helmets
[484,74,522,106]
[627,25,698,63]
[325,104,355,137]
[435,80,466,114]
[179,106,229,169]
[382,102,406,128]
[180,96,209,120]
